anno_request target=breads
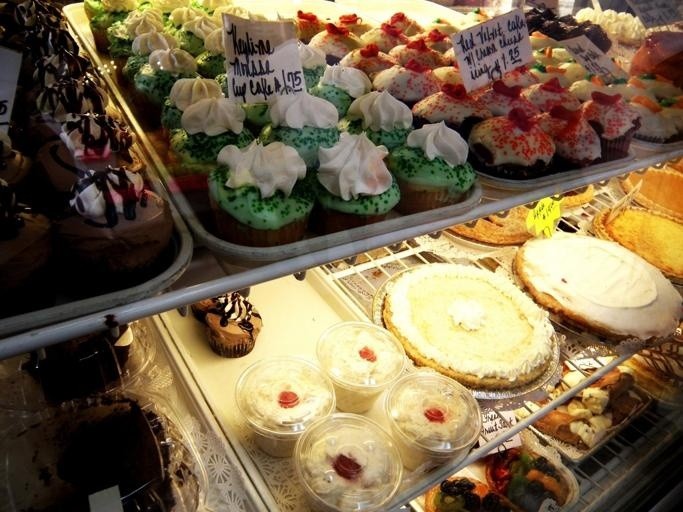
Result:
[524,369,652,462]
[596,205,683,284]
[558,185,595,208]
[619,340,683,402]
[444,202,539,247]
[619,168,683,219]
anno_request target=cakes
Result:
[316,321,410,391]
[414,66,642,179]
[432,10,491,35]
[20,324,142,400]
[22,396,184,511]
[284,10,427,97]
[190,289,263,359]
[528,29,554,52]
[629,31,682,77]
[514,231,683,341]
[530,46,599,105]
[576,6,648,46]
[296,412,403,512]
[383,264,552,388]
[237,359,334,439]
[524,4,612,52]
[388,372,481,459]
[600,69,683,145]
[426,28,470,68]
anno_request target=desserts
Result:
[84,0,476,247]
[1,1,170,280]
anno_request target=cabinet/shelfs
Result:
[0,145,682,512]
